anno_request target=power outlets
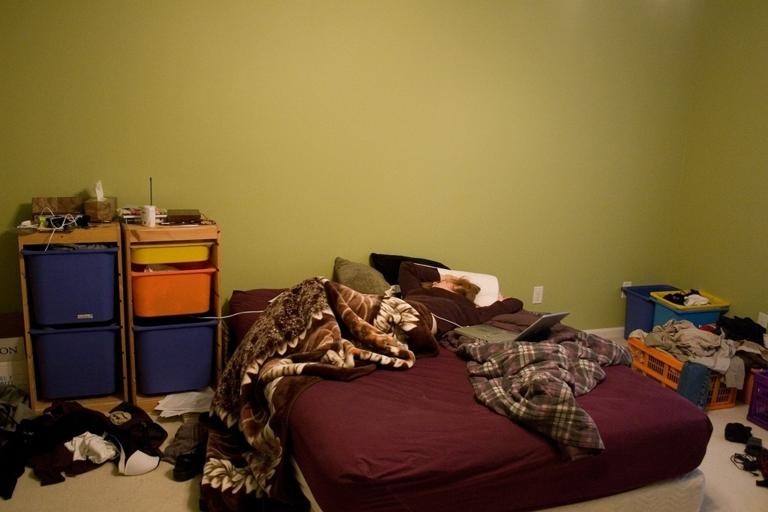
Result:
[532,286,545,304]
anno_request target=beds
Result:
[228,289,713,512]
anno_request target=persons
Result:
[395,262,524,357]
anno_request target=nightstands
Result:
[16,216,131,408]
[123,215,223,408]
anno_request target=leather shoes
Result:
[173,449,206,481]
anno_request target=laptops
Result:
[453,311,571,345]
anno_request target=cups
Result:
[141,205,156,227]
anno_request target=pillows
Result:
[334,256,391,295]
[417,262,500,308]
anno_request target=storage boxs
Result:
[22,246,118,326]
[131,241,214,264]
[746,371,768,433]
[29,325,120,402]
[622,284,680,340]
[648,288,730,332]
[741,367,765,405]
[133,315,218,395]
[626,333,739,412]
[131,263,216,317]
